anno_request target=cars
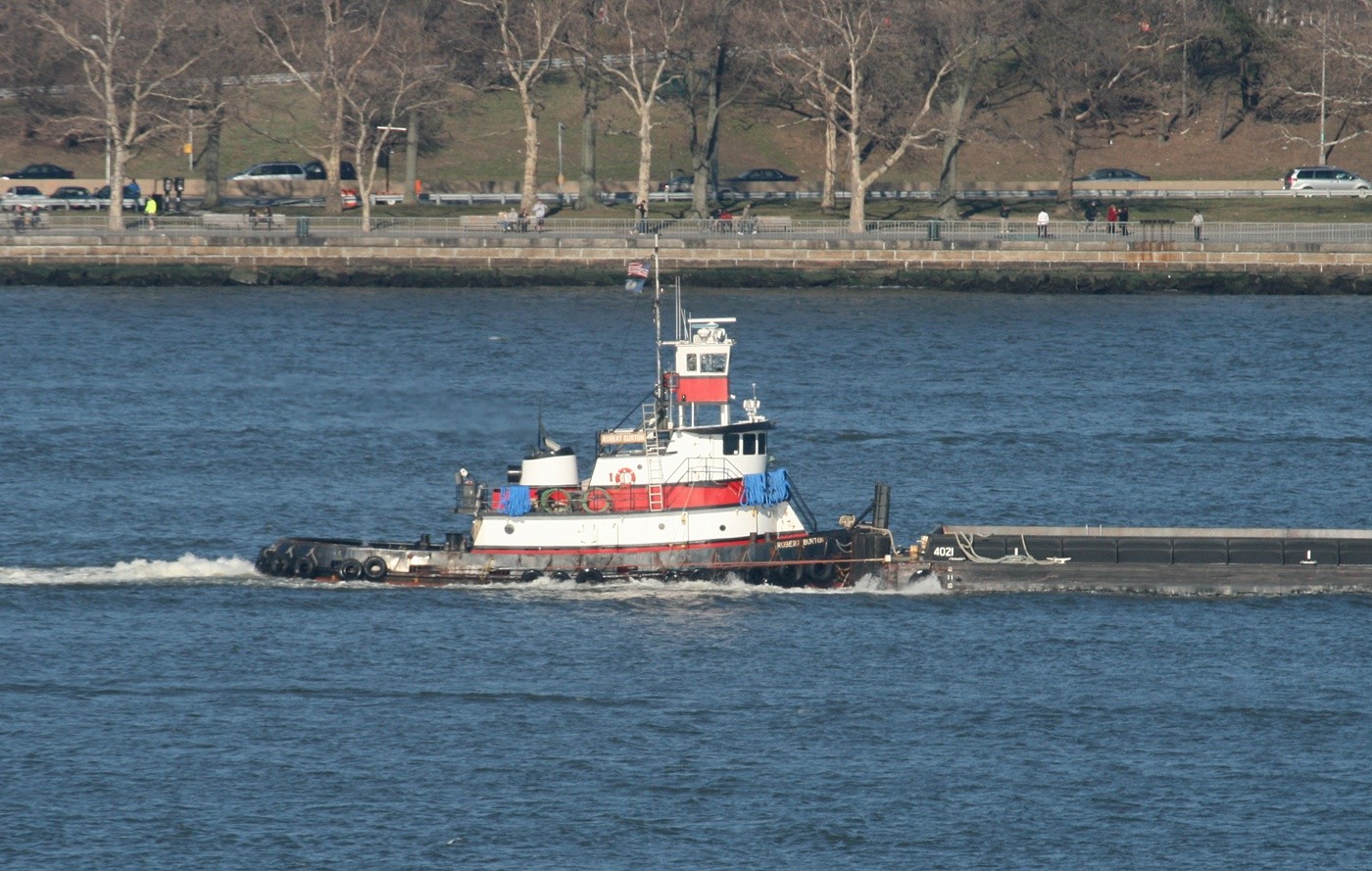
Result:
[0,162,75,180]
[659,174,739,203]
[95,184,144,211]
[302,159,356,180]
[719,167,801,197]
[229,161,308,181]
[2,185,43,196]
[49,186,93,211]
[1072,167,1151,182]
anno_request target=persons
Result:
[709,203,758,235]
[498,200,548,233]
[1000,203,1012,234]
[629,200,647,236]
[143,195,157,231]
[1106,201,1121,235]
[128,177,141,210]
[1084,200,1101,230]
[1191,210,1204,242]
[1036,207,1050,239]
[1114,199,1129,236]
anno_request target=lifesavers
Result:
[575,568,604,584]
[807,558,834,582]
[744,568,768,585]
[717,568,741,584]
[254,557,269,574]
[662,571,678,583]
[778,565,800,585]
[520,570,543,583]
[615,467,636,486]
[271,553,291,577]
[364,556,386,582]
[339,558,362,582]
[549,571,569,581]
[294,557,316,579]
[908,569,932,587]
[688,569,712,583]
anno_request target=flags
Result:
[625,260,653,294]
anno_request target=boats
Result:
[249,228,903,595]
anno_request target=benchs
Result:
[723,216,791,232]
[203,213,286,231]
[460,215,538,233]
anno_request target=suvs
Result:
[1282,165,1372,191]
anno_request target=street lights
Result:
[557,120,567,207]
[89,33,125,186]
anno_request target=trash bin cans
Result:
[928,223,940,240]
[297,216,309,236]
